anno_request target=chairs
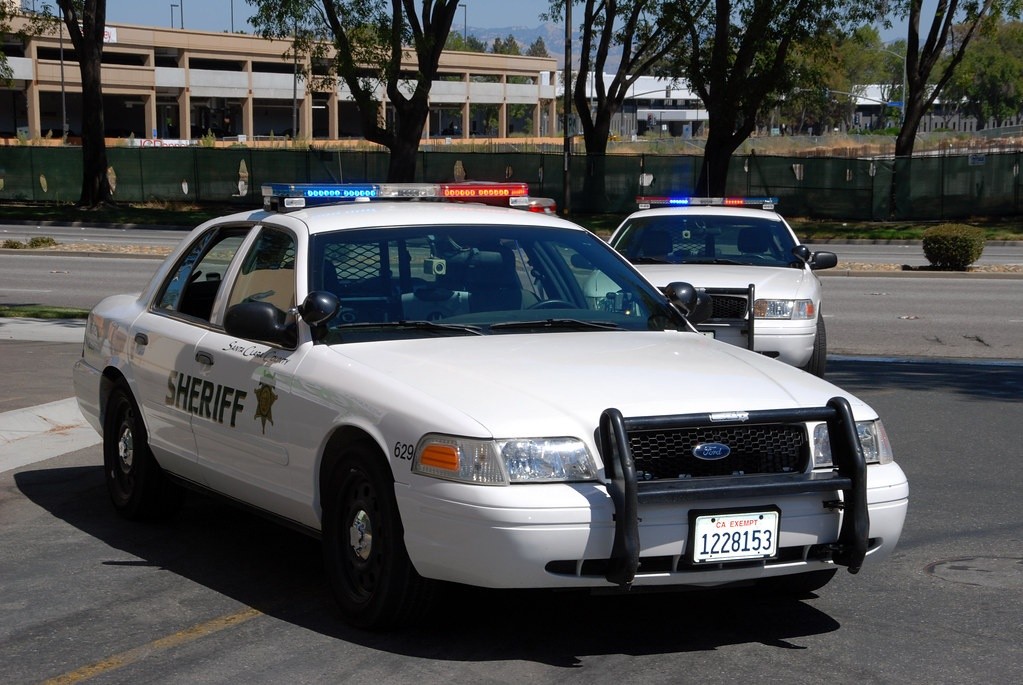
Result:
[283,258,351,329]
[737,227,776,260]
[465,245,541,312]
[632,228,674,260]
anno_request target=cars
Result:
[568,195,839,380]
[73,180,910,635]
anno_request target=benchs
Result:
[179,268,429,324]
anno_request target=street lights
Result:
[170,4,180,29]
[864,48,906,128]
[458,3,468,44]
[659,111,667,138]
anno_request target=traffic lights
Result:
[648,113,653,125]
[652,114,657,126]
[823,87,829,98]
